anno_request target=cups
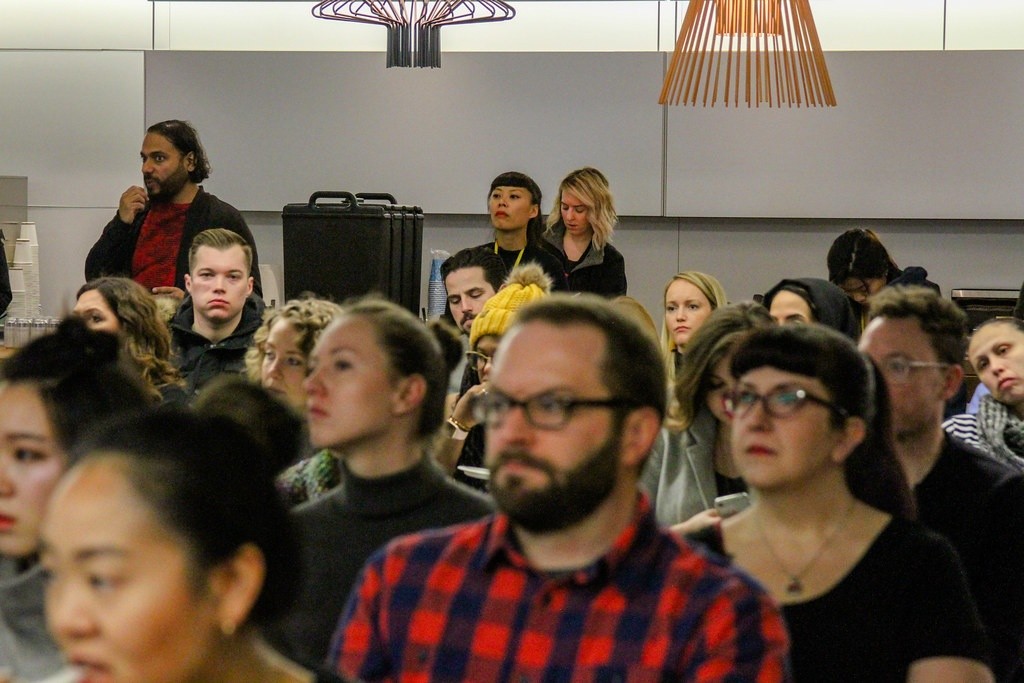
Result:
[13,239,33,317]
[7,268,26,318]
[428,259,447,317]
[20,222,39,319]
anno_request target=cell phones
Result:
[715,493,750,519]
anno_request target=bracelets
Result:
[450,416,472,432]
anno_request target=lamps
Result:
[659,0,839,107]
[311,0,515,70]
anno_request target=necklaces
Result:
[749,507,852,597]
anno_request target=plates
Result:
[457,464,491,480]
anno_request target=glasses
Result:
[471,389,647,430]
[875,356,956,385]
[465,348,496,371]
[722,386,850,419]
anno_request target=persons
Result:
[659,270,726,378]
[284,299,495,661]
[439,245,507,394]
[324,290,790,683]
[434,262,553,480]
[941,316,1024,473]
[73,276,187,415]
[43,373,348,683]
[245,298,344,411]
[0,315,149,683]
[479,172,569,294]
[637,300,776,529]
[84,120,263,303]
[686,322,996,683]
[759,277,856,339]
[827,228,942,334]
[164,228,264,398]
[542,167,628,301]
[857,285,1024,648]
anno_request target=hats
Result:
[470,262,553,351]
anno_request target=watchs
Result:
[444,420,469,441]
